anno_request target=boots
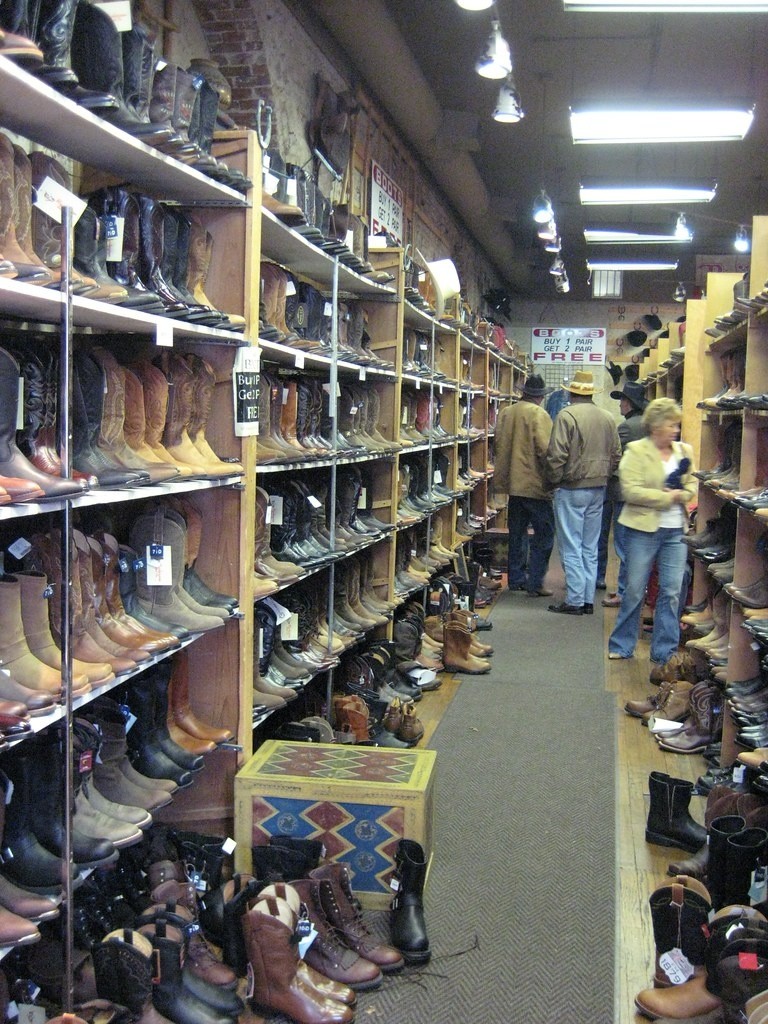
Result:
[0,0,768,1024]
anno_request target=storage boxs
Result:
[483,526,536,572]
[232,741,440,909]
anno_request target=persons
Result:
[601,381,651,608]
[542,371,622,615]
[488,371,554,597]
[609,395,697,669]
[558,479,612,591]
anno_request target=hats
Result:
[560,370,604,395]
[415,245,462,321]
[514,374,556,397]
[610,381,652,411]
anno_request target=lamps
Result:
[672,281,688,303]
[554,269,573,293]
[564,92,758,147]
[733,223,750,254]
[491,75,526,124]
[549,253,567,277]
[583,253,682,270]
[542,233,562,253]
[532,187,556,224]
[472,20,516,81]
[562,0,768,13]
[579,219,694,246]
[580,182,720,208]
[673,211,692,240]
[536,216,558,239]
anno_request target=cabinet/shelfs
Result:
[0,53,768,1024]
